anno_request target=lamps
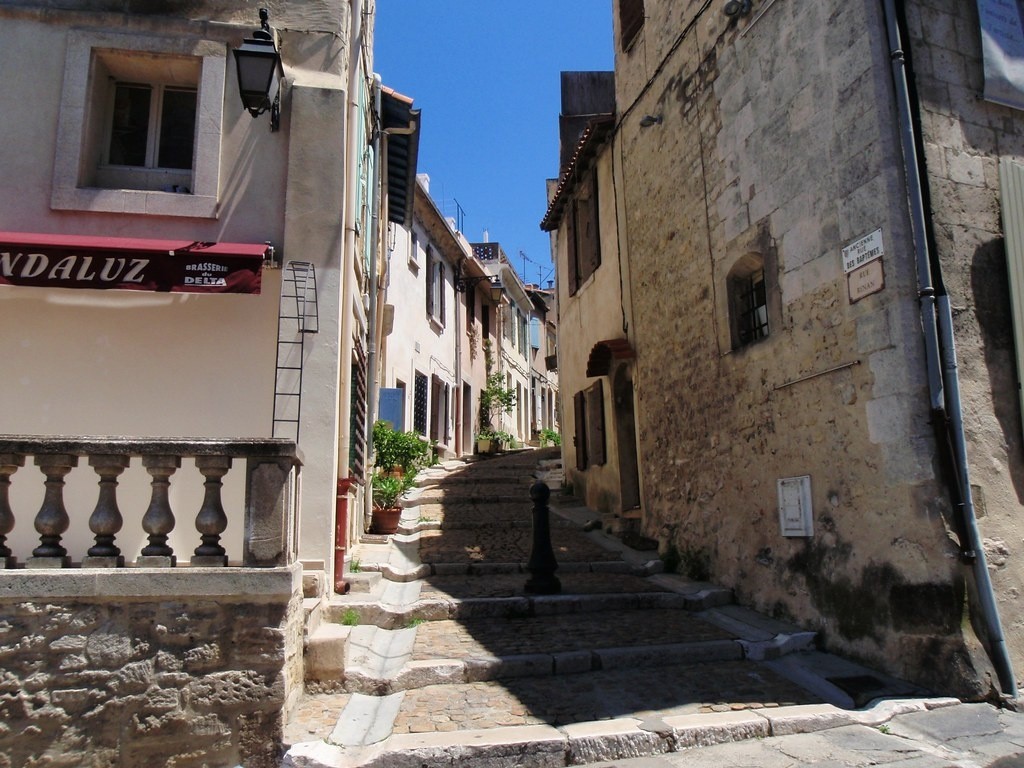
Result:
[456,273,508,308]
[639,113,663,128]
[231,7,286,133]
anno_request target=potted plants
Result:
[528,429,562,448]
[369,421,443,535]
[475,368,520,454]
